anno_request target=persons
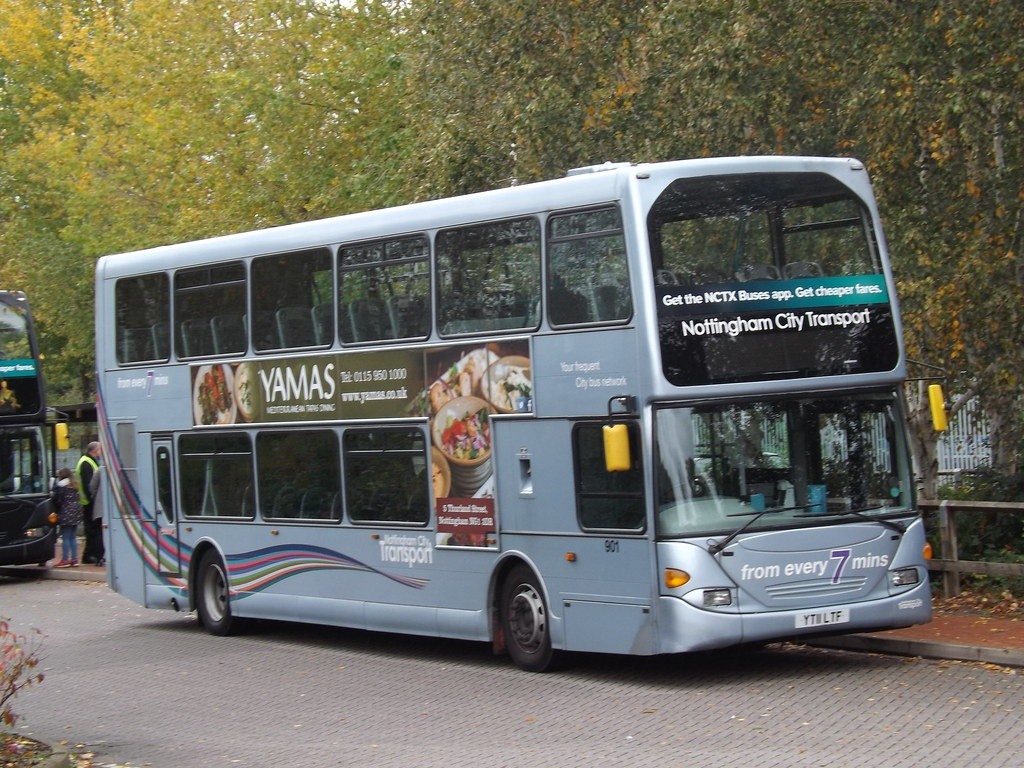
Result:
[51,467,83,568]
[88,464,106,567]
[74,441,106,564]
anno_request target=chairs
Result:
[242,476,427,518]
[123,261,853,360]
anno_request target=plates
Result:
[431,396,498,468]
[479,355,531,413]
[193,364,237,425]
[431,446,452,515]
[234,362,255,419]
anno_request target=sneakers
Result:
[53,561,71,568]
[82,555,105,563]
[71,559,79,567]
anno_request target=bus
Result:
[0,289,72,573]
[95,155,951,673]
